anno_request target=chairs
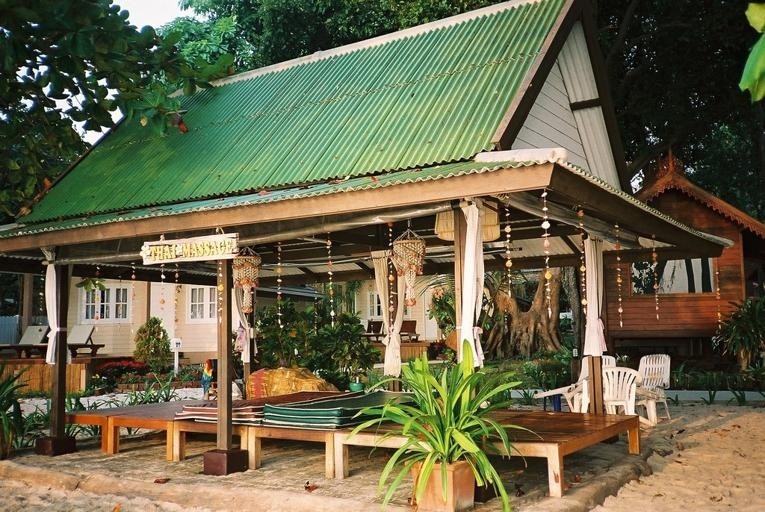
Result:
[562,354,672,440]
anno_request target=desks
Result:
[65,400,204,463]
[173,421,247,463]
[247,426,333,478]
[333,409,641,499]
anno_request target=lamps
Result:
[392,218,426,307]
[232,245,262,314]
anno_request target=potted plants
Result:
[346,339,544,512]
